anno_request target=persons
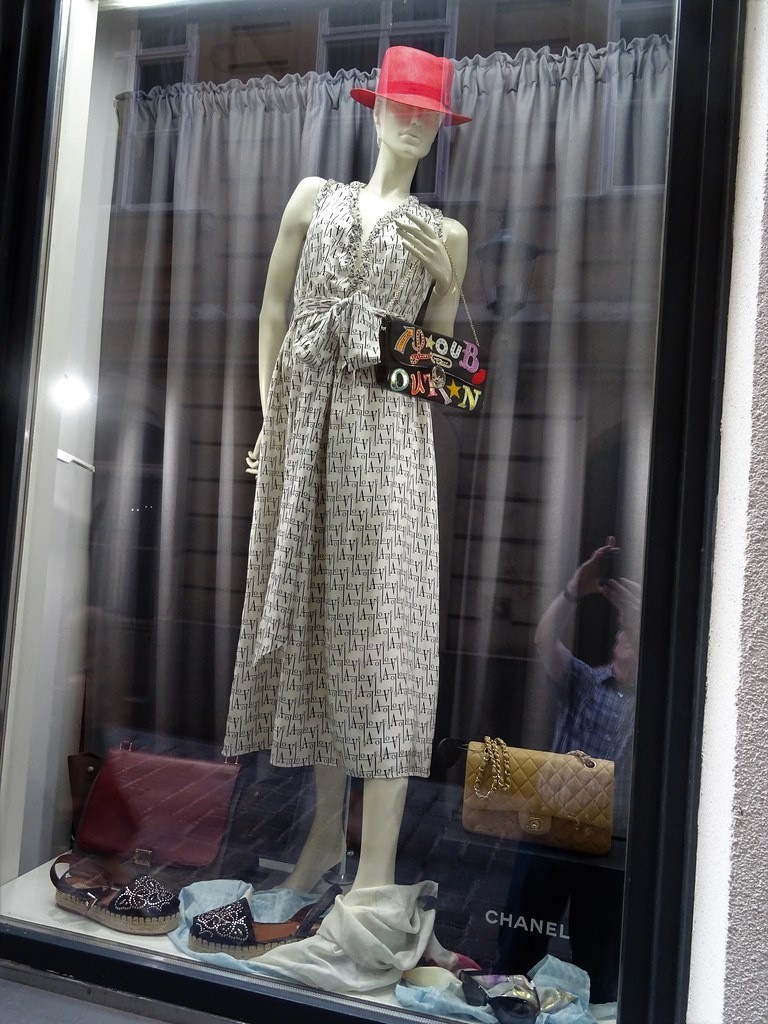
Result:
[220,47,475,898]
[495,537,642,1009]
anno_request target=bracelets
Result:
[562,586,581,603]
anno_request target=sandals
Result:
[187,883,343,962]
[51,852,183,934]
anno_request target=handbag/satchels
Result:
[374,249,490,416]
[461,735,617,855]
[76,741,243,869]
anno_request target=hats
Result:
[349,43,475,126]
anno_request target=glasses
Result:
[460,970,541,1024]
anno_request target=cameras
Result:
[597,550,620,586]
